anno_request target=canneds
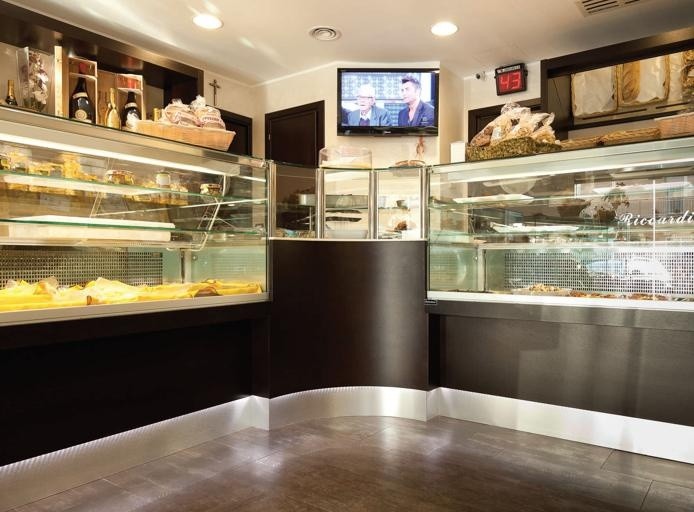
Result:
[200,182,222,206]
[156,172,190,205]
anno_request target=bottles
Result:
[104,87,121,130]
[69,63,95,124]
[199,183,222,204]
[104,169,135,199]
[155,171,171,190]
[153,107,161,122]
[120,77,141,127]
[4,79,19,107]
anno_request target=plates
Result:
[592,182,693,199]
[509,286,573,296]
[452,193,535,209]
[492,224,581,232]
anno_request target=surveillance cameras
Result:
[476,71,486,82]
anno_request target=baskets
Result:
[559,136,601,152]
[137,120,237,151]
[602,127,659,147]
[656,112,694,140]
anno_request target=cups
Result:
[378,195,386,208]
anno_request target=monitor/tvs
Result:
[336,68,439,137]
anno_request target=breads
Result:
[394,159,424,167]
[527,281,694,302]
[1,276,263,313]
[471,124,556,149]
[163,97,226,130]
[0,144,155,204]
[681,49,694,100]
[621,61,641,99]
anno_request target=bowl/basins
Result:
[329,229,369,239]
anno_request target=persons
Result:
[398,75,435,127]
[347,84,392,126]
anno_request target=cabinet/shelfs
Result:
[427,136,694,313]
[268,158,426,241]
[1,102,276,326]
